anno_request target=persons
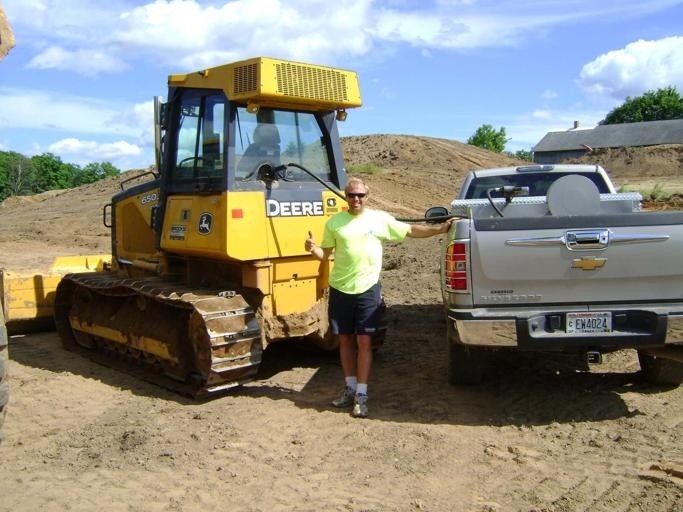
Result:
[304,176,461,418]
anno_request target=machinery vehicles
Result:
[2,56,390,399]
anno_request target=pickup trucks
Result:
[424,162,683,388]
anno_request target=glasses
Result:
[347,193,365,198]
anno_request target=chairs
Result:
[237,124,279,176]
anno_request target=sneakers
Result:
[332,386,369,417]
